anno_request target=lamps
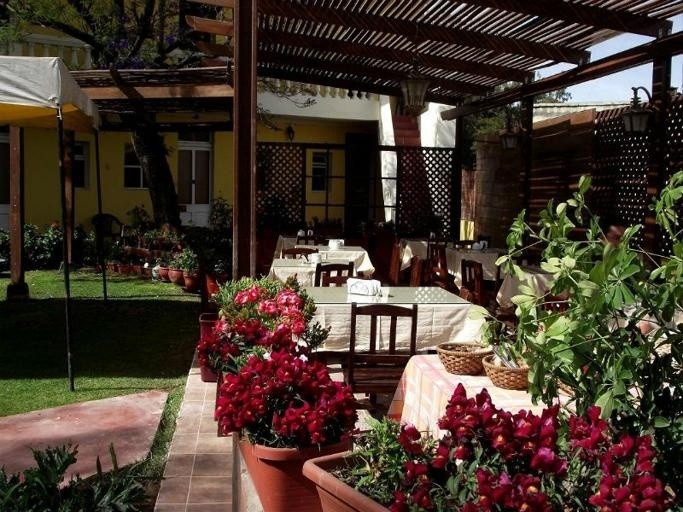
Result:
[397,25,431,117]
[620,85,659,139]
[499,121,521,155]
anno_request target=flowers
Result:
[390,382,669,512]
[189,279,359,443]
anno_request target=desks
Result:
[400,353,579,429]
[264,226,368,288]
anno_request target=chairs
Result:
[91,214,125,244]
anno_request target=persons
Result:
[591,217,630,268]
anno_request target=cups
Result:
[381,288,389,297]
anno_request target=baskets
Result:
[437,342,493,374]
[482,356,531,388]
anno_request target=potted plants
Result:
[107,225,200,290]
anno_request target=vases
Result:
[228,431,388,512]
